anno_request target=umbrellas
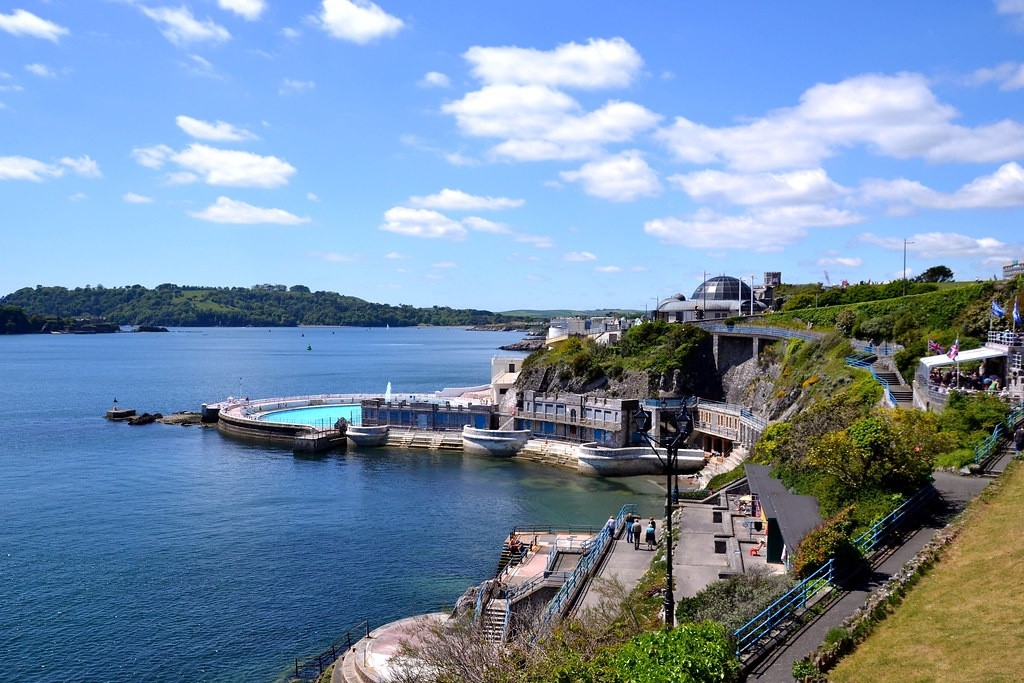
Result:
[740,495,751,501]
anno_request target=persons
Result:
[649,517,656,530]
[740,504,747,513]
[869,339,874,350]
[645,525,655,550]
[996,329,1022,346]
[931,366,1007,396]
[871,276,955,285]
[508,535,519,557]
[631,519,641,550]
[709,490,714,495]
[753,541,765,551]
[625,513,634,543]
[608,516,615,537]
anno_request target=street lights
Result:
[633,397,695,633]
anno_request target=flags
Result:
[929,340,945,354]
[1013,302,1021,324]
[947,342,959,361]
[992,300,1005,319]
